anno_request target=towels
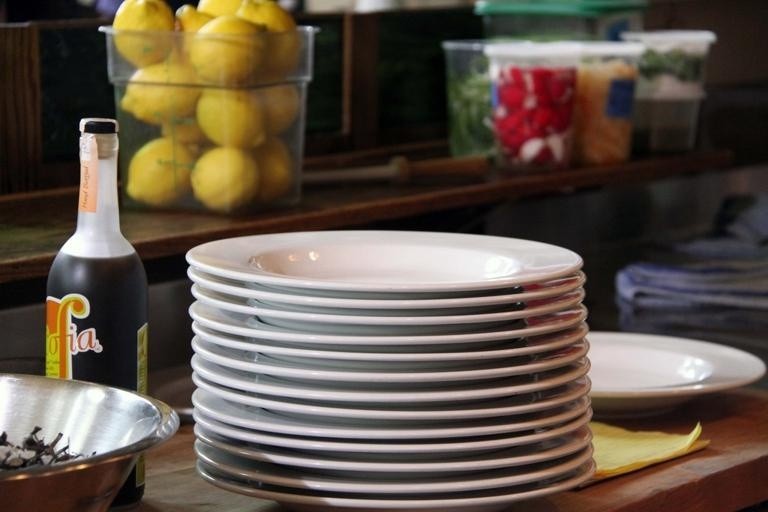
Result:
[614,196,768,315]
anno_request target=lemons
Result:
[111,0,305,215]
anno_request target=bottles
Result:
[43,115,151,510]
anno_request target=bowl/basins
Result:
[1,371,181,512]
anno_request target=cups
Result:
[439,26,717,171]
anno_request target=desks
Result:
[134,386,768,510]
[0,141,768,285]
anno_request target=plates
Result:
[583,328,767,418]
[186,227,598,511]
